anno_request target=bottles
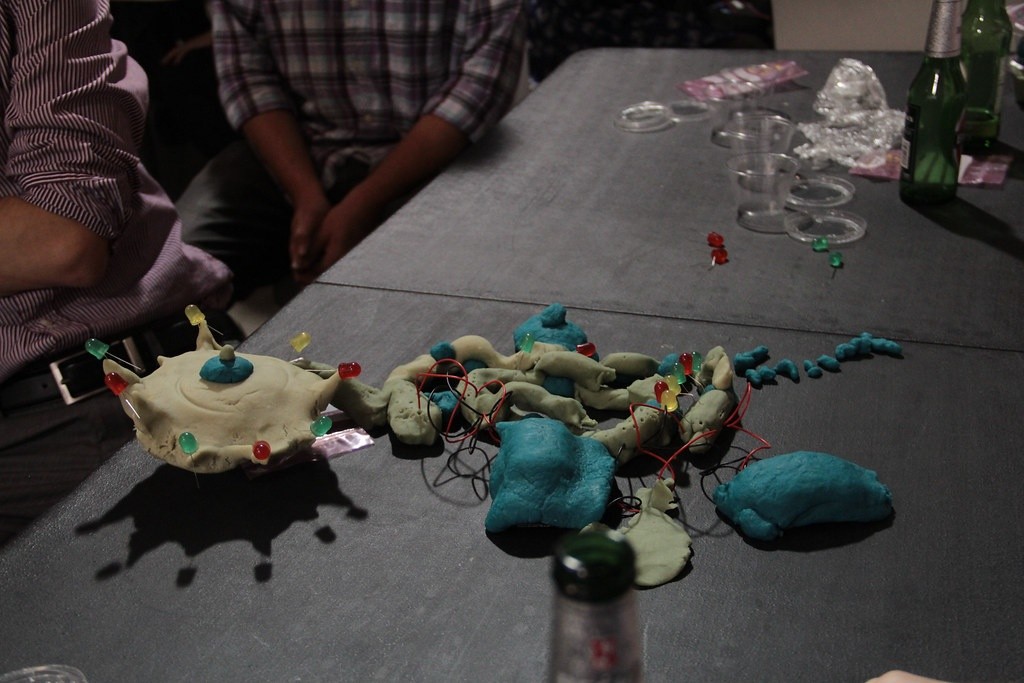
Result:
[899,0,966,209]
[547,534,645,682]
[961,0,1013,157]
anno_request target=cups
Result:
[703,81,757,147]
[725,153,798,216]
[725,116,797,157]
[0,664,88,683]
[731,64,778,119]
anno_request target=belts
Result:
[0,322,183,410]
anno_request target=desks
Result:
[0,47,1024,683]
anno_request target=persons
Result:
[175,0,532,301]
[0,0,247,552]
